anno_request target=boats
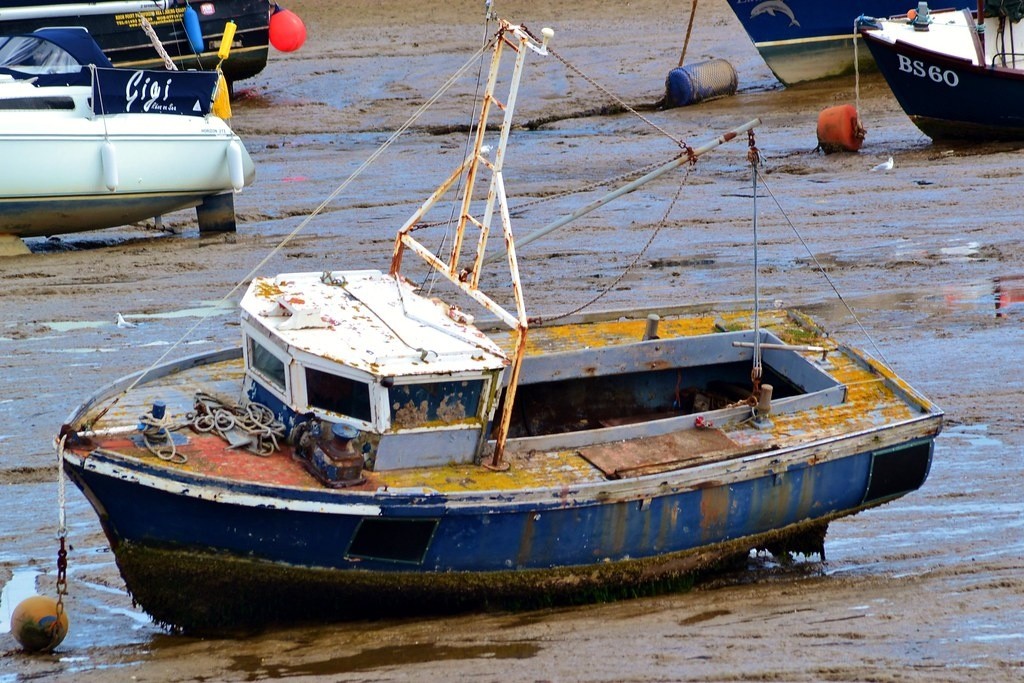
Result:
[0,0,269,237]
[861,3,1020,146]
[728,0,983,88]
[53,1,942,636]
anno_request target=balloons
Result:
[269,4,306,52]
